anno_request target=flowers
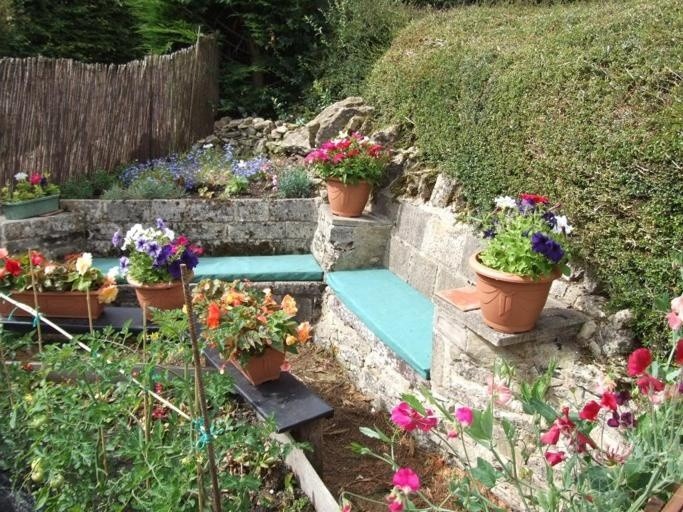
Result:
[0,168,61,202]
[336,288,683,512]
[467,190,575,284]
[306,127,393,189]
[191,276,309,372]
[0,248,118,304]
[111,217,204,287]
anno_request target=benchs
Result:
[90,253,322,325]
[313,267,434,411]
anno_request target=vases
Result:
[468,250,561,335]
[325,177,371,217]
[1,194,60,220]
[229,349,282,386]
[125,269,193,322]
[0,291,105,321]
